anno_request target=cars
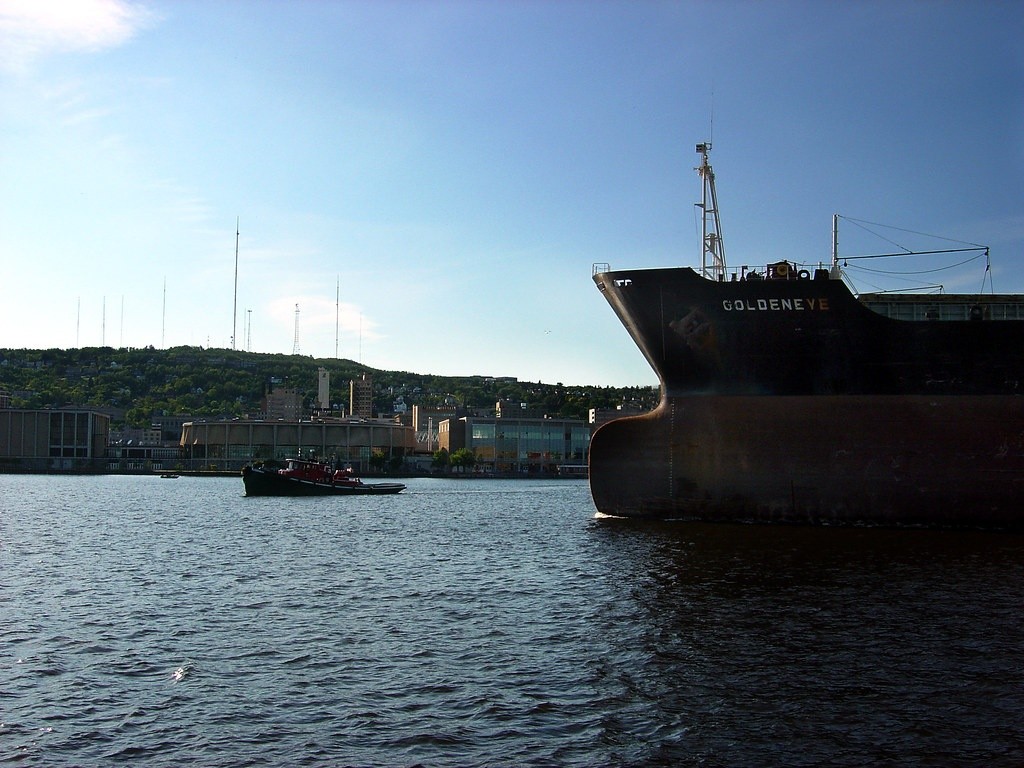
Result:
[416,468,430,473]
[430,468,446,475]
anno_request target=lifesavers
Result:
[969,305,984,320]
[777,264,788,276]
[797,270,811,281]
[549,464,556,471]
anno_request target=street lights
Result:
[547,432,551,461]
[526,432,529,458]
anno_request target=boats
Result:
[242,447,406,497]
[552,464,589,479]
[588,141,1024,521]
[161,473,179,478]
